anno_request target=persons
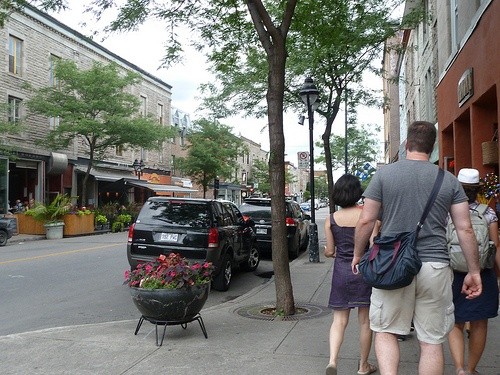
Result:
[351,121,482,375]
[3,199,29,214]
[325,168,500,375]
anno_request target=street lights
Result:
[297,75,324,264]
[132,158,145,206]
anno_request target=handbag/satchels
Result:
[358,231,422,290]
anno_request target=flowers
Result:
[121,253,216,292]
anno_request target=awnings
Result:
[77,166,198,196]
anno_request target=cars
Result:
[299,193,330,211]
[0,215,13,247]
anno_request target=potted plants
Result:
[16,202,47,235]
[95,215,108,226]
[65,208,96,236]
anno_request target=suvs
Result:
[126,196,261,291]
[238,199,314,260]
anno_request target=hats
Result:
[457,168,485,190]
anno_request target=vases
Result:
[129,282,211,325]
[44,219,64,239]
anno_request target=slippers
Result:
[326,365,337,375]
[356,364,377,375]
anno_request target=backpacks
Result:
[447,203,496,272]
